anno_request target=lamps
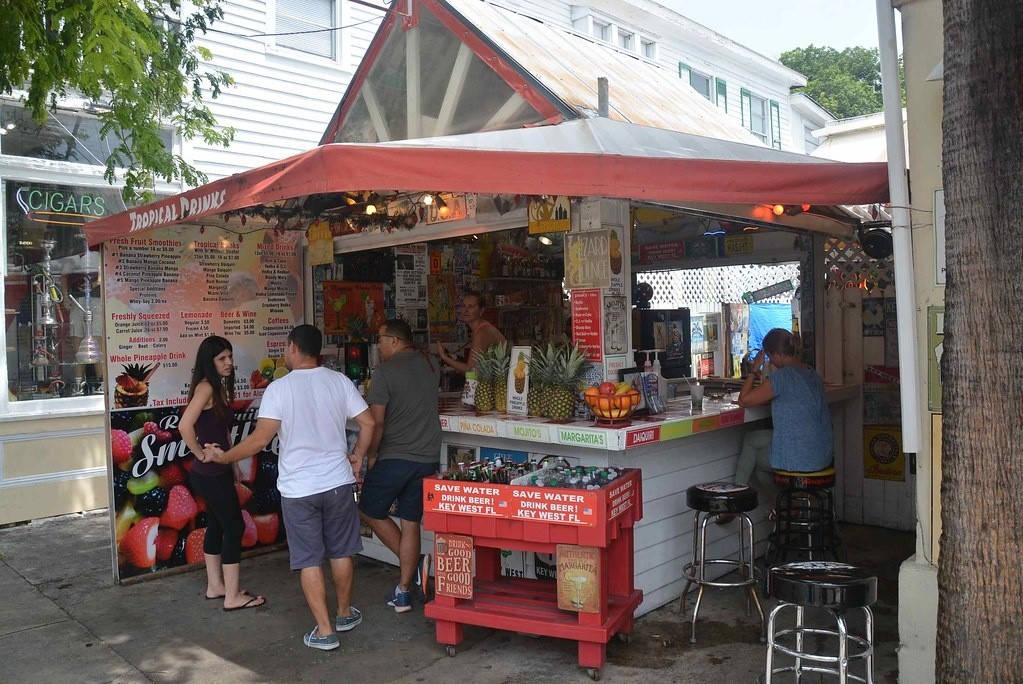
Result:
[347,217,359,231]
[538,233,559,246]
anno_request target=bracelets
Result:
[751,371,757,376]
[198,453,205,462]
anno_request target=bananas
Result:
[614,382,632,394]
[594,407,628,417]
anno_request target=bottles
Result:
[351,366,372,401]
[428,458,624,515]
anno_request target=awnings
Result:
[83,116,912,251]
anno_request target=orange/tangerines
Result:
[585,388,640,409]
[259,358,289,379]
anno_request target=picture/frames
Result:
[619,368,650,416]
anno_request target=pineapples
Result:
[113,363,150,409]
[610,228,622,274]
[472,341,511,413]
[515,351,526,394]
[522,341,593,421]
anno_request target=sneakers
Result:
[413,553,432,604]
[385,583,412,612]
[336,606,362,631]
[304,625,340,650]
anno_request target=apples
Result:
[599,382,616,394]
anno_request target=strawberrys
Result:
[250,370,270,389]
[111,422,278,568]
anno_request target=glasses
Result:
[375,333,405,344]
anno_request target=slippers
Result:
[764,509,799,520]
[223,597,265,611]
[205,591,249,600]
[715,515,735,525]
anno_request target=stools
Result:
[680,467,877,684]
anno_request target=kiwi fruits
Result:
[262,367,274,379]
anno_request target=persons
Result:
[557,320,572,346]
[357,319,442,613]
[208,324,376,649]
[717,329,834,524]
[436,293,507,372]
[178,337,267,612]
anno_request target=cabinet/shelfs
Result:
[480,277,560,344]
[423,466,643,681]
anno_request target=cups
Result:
[690,385,706,411]
